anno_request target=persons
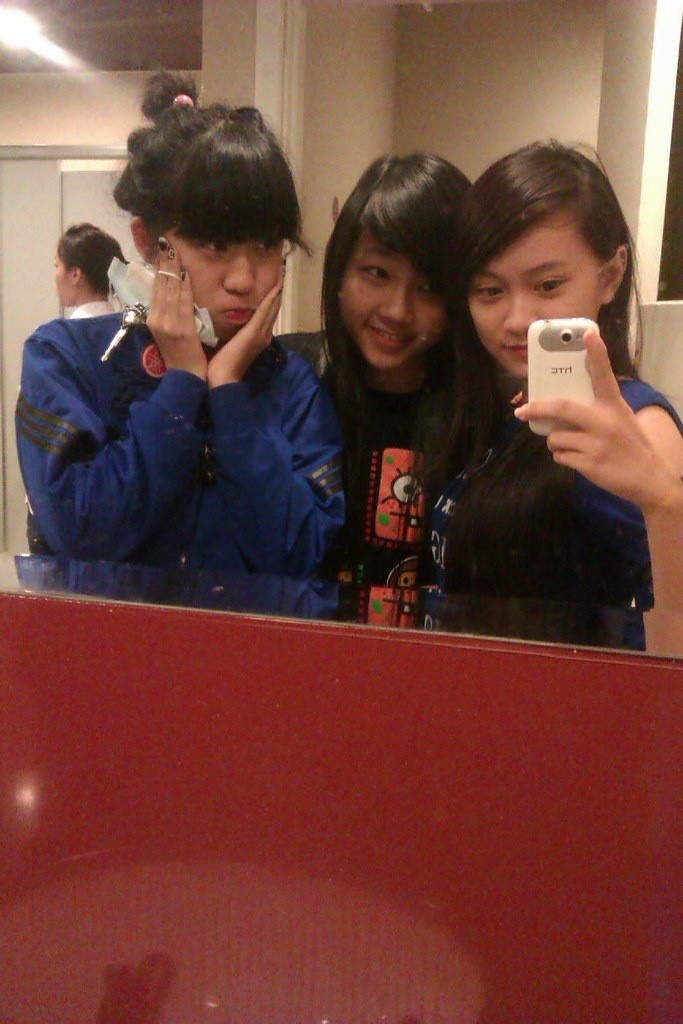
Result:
[14,71,346,580]
[391,142,683,611]
[24,222,129,556]
[274,152,508,591]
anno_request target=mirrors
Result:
[0,0,682,664]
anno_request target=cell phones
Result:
[527,318,601,438]
